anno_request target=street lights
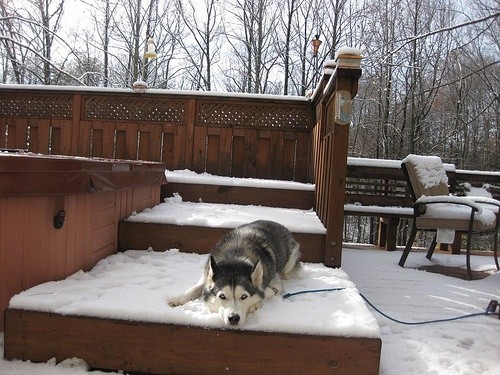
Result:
[311,34,322,82]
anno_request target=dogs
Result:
[168,220,302,326]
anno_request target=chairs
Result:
[398,154,500,279]
